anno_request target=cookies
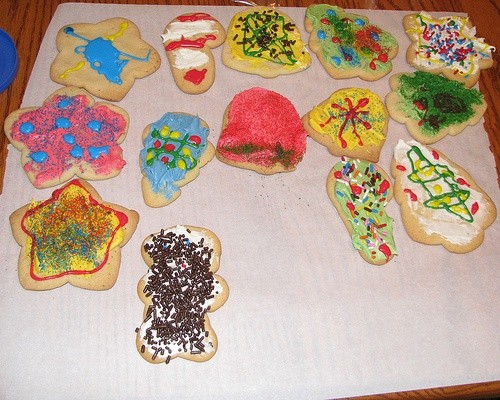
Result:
[49,14,159,103]
[220,7,312,80]
[3,85,131,188]
[216,86,306,175]
[300,88,391,163]
[10,179,141,292]
[161,12,226,95]
[385,70,488,143]
[134,224,230,363]
[326,159,400,266]
[404,11,496,88]
[139,112,216,210]
[391,139,497,254]
[304,3,399,81]
